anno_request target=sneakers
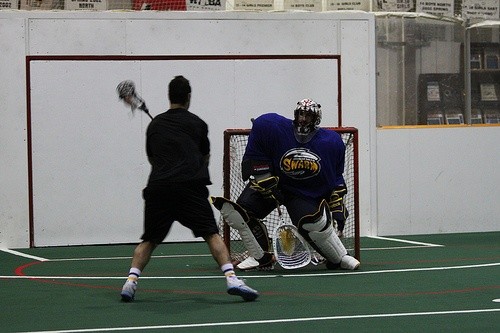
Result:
[226,280,258,300]
[120,280,137,302]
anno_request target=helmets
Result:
[293,98,321,135]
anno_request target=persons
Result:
[210,98,361,269]
[120,74,259,301]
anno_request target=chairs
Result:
[470,54,498,69]
[426,113,499,124]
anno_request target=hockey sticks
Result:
[273,201,311,269]
[115,80,154,120]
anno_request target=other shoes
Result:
[246,261,274,272]
[340,255,359,270]
